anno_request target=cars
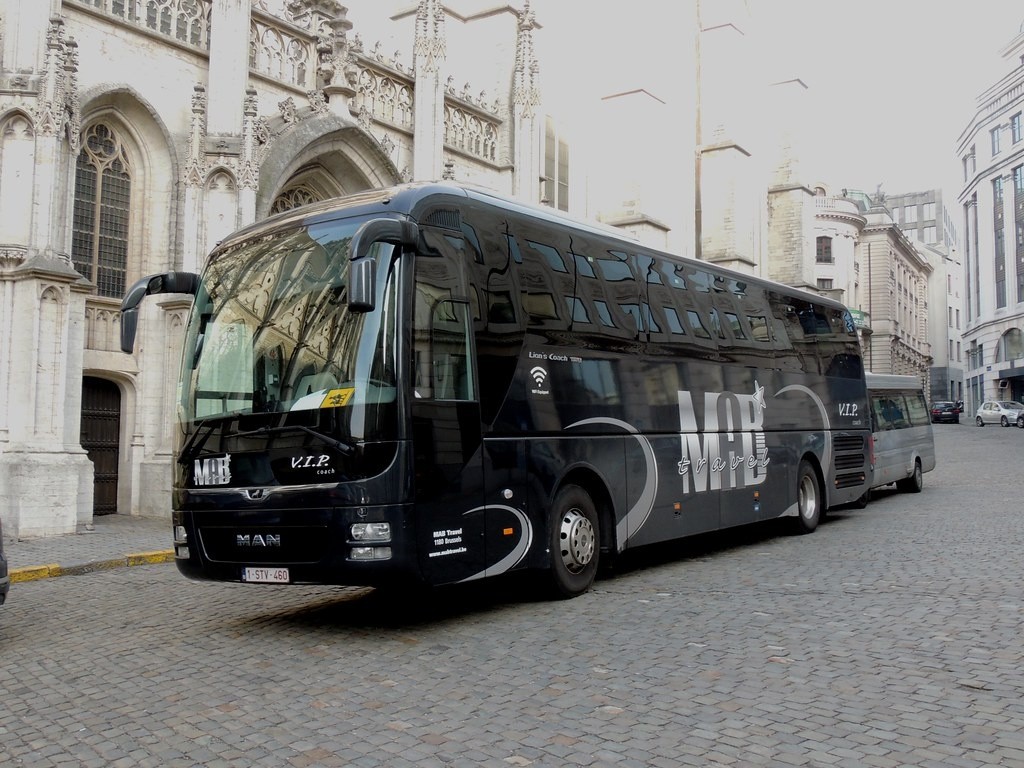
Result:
[974,400,1024,429]
[928,400,961,424]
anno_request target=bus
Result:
[117,180,874,616]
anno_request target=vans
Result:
[861,373,938,495]
